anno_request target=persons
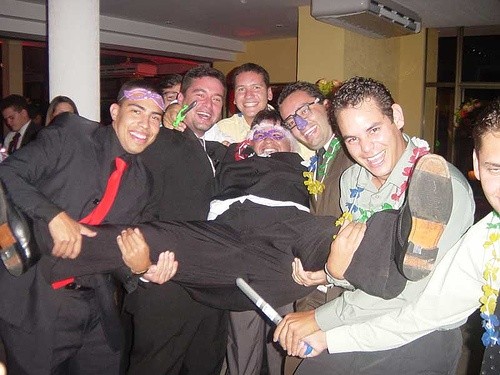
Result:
[26,100,47,126]
[0,94,41,164]
[0,108,455,302]
[160,63,316,375]
[0,75,166,375]
[124,67,227,375]
[44,95,80,126]
[158,71,182,107]
[275,80,357,375]
[270,76,476,375]
[287,106,500,375]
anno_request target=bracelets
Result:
[131,260,152,275]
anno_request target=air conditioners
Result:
[100,62,158,77]
[310,0,422,41]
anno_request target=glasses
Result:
[281,99,323,130]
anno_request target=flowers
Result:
[456,99,486,129]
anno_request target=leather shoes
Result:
[394,154,454,281]
[0,179,43,277]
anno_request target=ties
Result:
[7,132,21,155]
[51,157,127,289]
[315,147,326,181]
